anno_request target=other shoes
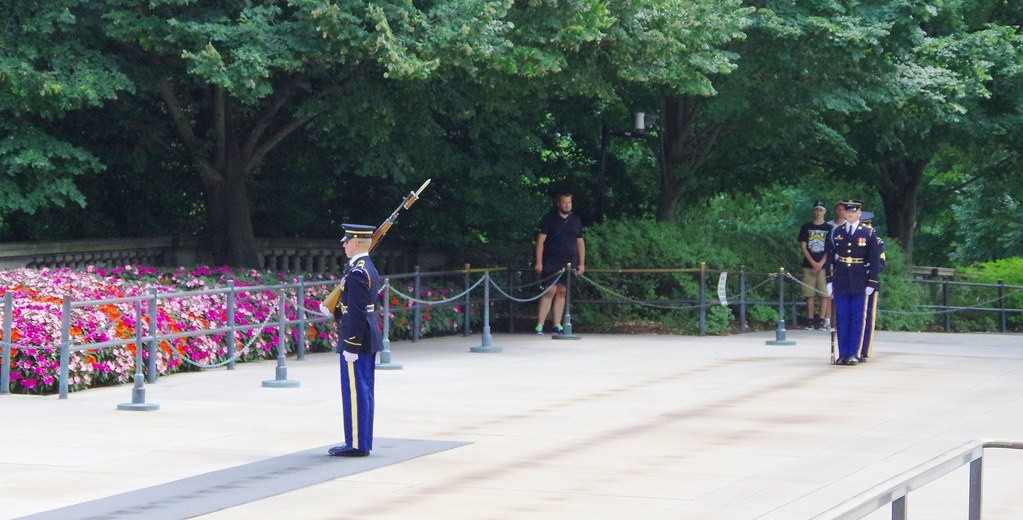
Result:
[804,318,815,330]
[328,444,370,456]
[836,355,859,365]
[819,318,827,331]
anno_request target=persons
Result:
[319,223,383,457]
[798,199,886,365]
[535,190,585,335]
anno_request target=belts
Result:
[838,256,864,263]
[340,303,375,314]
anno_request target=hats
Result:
[813,202,827,211]
[842,198,864,211]
[835,200,847,210]
[861,211,874,225]
[340,223,376,242]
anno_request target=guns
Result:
[829,263,836,365]
[319,178,432,318]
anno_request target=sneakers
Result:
[552,324,564,335]
[535,323,544,334]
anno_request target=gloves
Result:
[320,305,334,320]
[342,350,358,362]
[865,286,875,295]
[826,283,833,297]
